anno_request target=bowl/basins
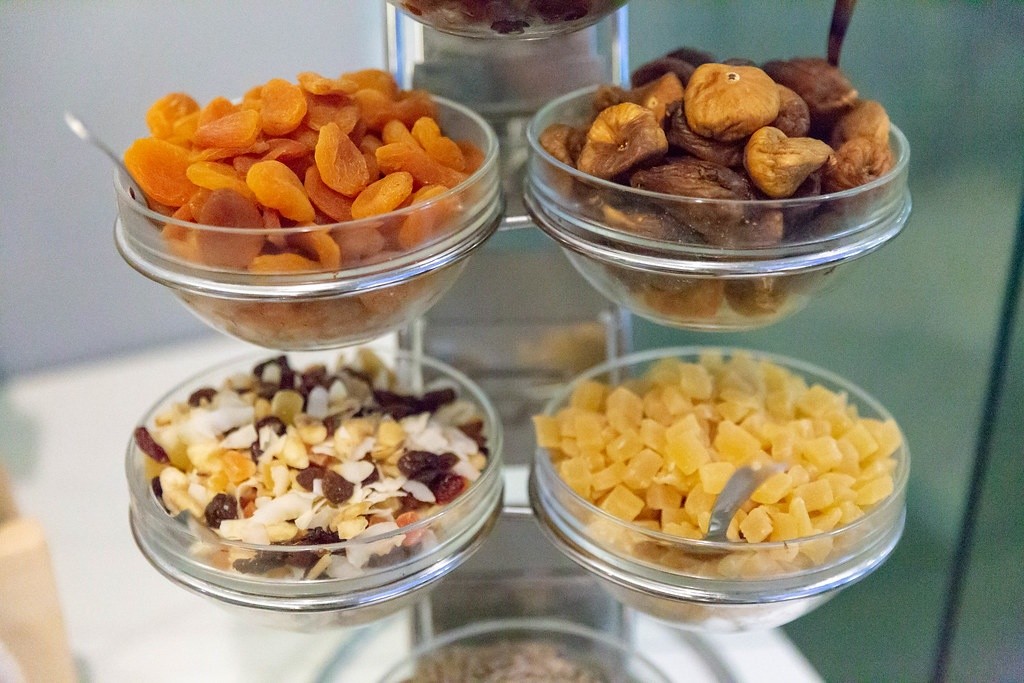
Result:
[527,343,912,632]
[383,0,630,43]
[370,618,675,683]
[112,85,505,352]
[127,349,504,634]
[519,86,916,335]
